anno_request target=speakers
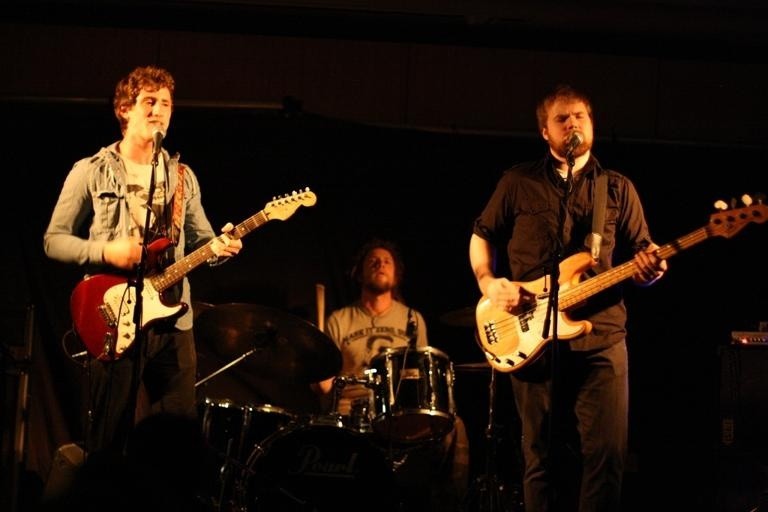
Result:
[715,349,768,458]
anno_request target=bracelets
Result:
[476,266,492,280]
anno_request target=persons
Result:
[468,86,669,512]
[310,244,470,478]
[43,66,244,435]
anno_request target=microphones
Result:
[149,125,168,165]
[562,130,584,158]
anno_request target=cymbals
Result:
[438,305,478,328]
[192,304,342,386]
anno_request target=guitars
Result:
[70,187,319,362]
[476,193,765,373]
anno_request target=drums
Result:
[234,404,296,462]
[200,398,244,461]
[370,344,455,447]
[230,411,405,512]
